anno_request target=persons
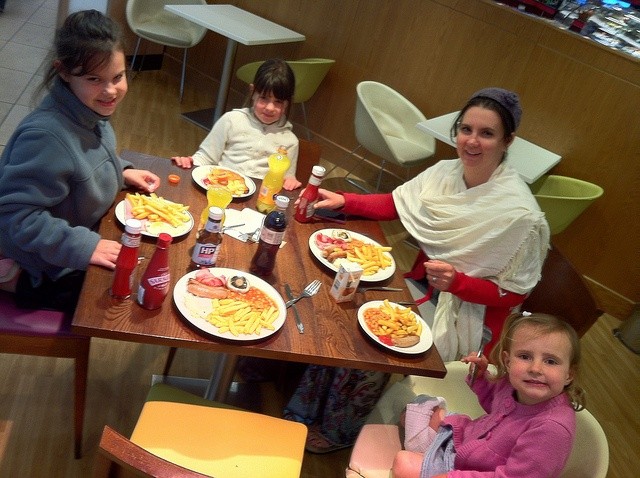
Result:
[389,307,587,477]
[280,87,545,450]
[170,57,302,191]
[1,7,161,316]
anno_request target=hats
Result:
[471,88,523,132]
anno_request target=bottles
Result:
[569,11,589,34]
[137,232,173,311]
[111,219,141,299]
[256,145,291,213]
[294,166,326,223]
[189,206,224,270]
[196,177,233,242]
[249,195,289,277]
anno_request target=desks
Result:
[417,109,562,185]
[163,1,306,133]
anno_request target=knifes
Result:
[284,284,304,334]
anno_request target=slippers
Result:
[305,425,355,454]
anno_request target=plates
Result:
[115,197,195,237]
[173,267,288,343]
[357,300,434,355]
[308,227,397,283]
[192,165,257,199]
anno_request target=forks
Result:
[356,286,402,293]
[469,325,493,386]
[285,279,322,309]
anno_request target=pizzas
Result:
[216,168,243,182]
[363,307,387,335]
[345,239,367,249]
[228,285,280,311]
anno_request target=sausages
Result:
[188,284,228,299]
[328,251,348,263]
[322,244,335,258]
[189,277,227,289]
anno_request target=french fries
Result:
[348,245,394,279]
[125,192,193,228]
[227,178,248,197]
[205,297,280,337]
[379,298,422,340]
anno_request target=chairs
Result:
[532,174,606,237]
[519,243,606,340]
[321,80,436,195]
[234,57,335,141]
[97,380,309,478]
[299,141,320,185]
[343,358,611,478]
[126,1,207,98]
[0,258,92,461]
